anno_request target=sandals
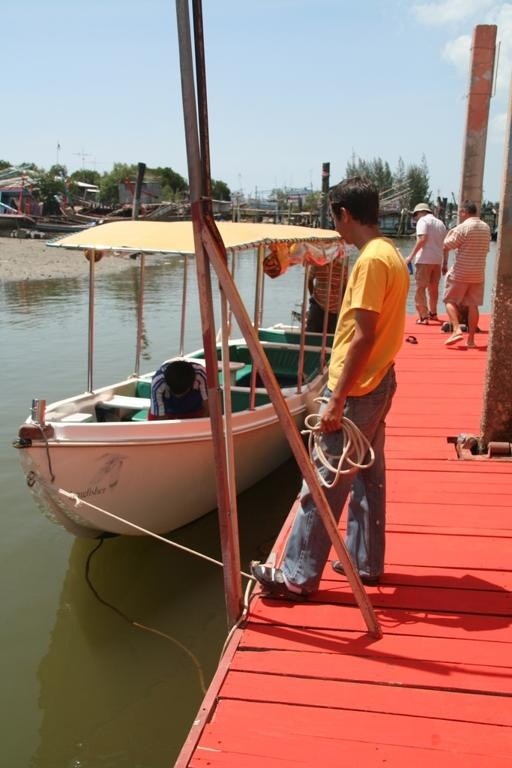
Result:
[251,560,311,605]
[331,557,382,586]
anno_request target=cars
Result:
[0,199,37,234]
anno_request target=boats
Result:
[10,219,345,539]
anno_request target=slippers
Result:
[405,335,419,343]
[444,334,464,346]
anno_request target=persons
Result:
[440,197,493,348]
[302,255,348,335]
[244,173,413,603]
[404,201,449,325]
[145,356,226,421]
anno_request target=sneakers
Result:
[415,316,430,325]
[428,312,439,321]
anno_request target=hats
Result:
[410,201,434,214]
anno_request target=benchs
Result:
[123,330,334,421]
